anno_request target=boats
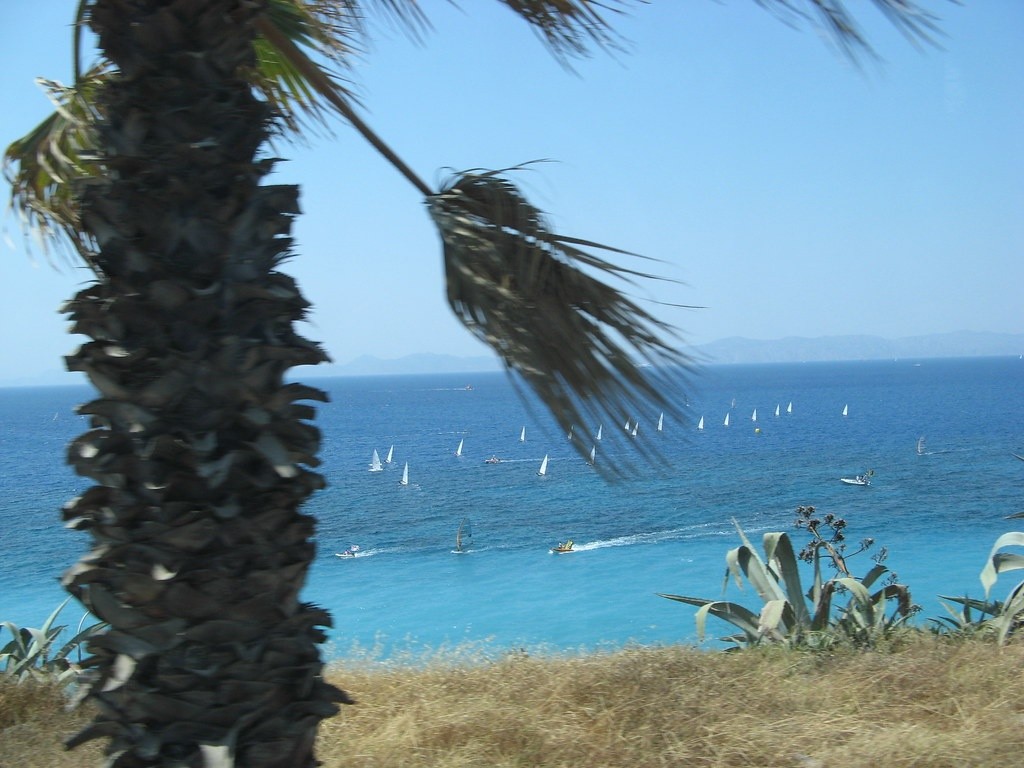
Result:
[552,540,574,553]
[335,552,355,557]
[839,472,870,485]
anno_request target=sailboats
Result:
[367,395,850,487]
[453,519,474,555]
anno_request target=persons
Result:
[345,549,350,555]
[858,474,868,483]
[558,542,562,548]
